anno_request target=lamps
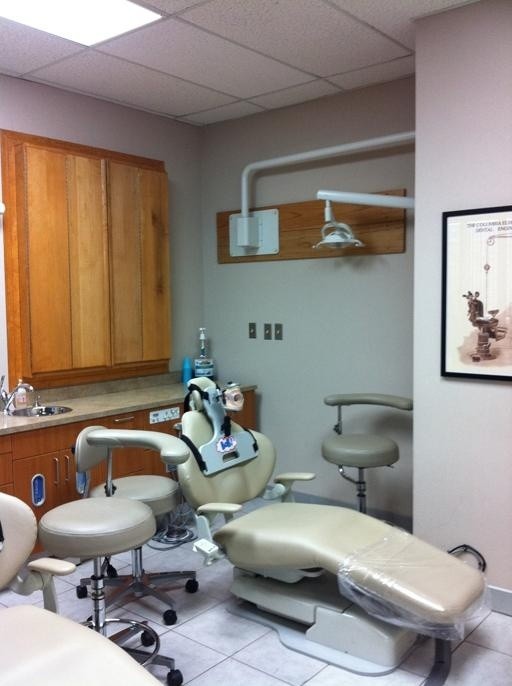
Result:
[311,189,414,248]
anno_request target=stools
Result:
[38,496,184,685]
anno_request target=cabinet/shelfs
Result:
[10,420,102,553]
[110,410,151,478]
[20,140,172,373]
[0,435,15,496]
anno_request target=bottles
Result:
[199,326,209,358]
[182,356,193,385]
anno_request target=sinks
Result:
[11,403,72,418]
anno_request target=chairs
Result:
[322,393,413,513]
[179,376,483,675]
[75,424,197,623]
[0,491,164,686]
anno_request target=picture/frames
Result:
[440,205,512,381]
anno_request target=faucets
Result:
[0,375,34,415]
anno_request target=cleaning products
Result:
[195,326,215,384]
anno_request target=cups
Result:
[195,359,214,378]
[14,392,30,408]
